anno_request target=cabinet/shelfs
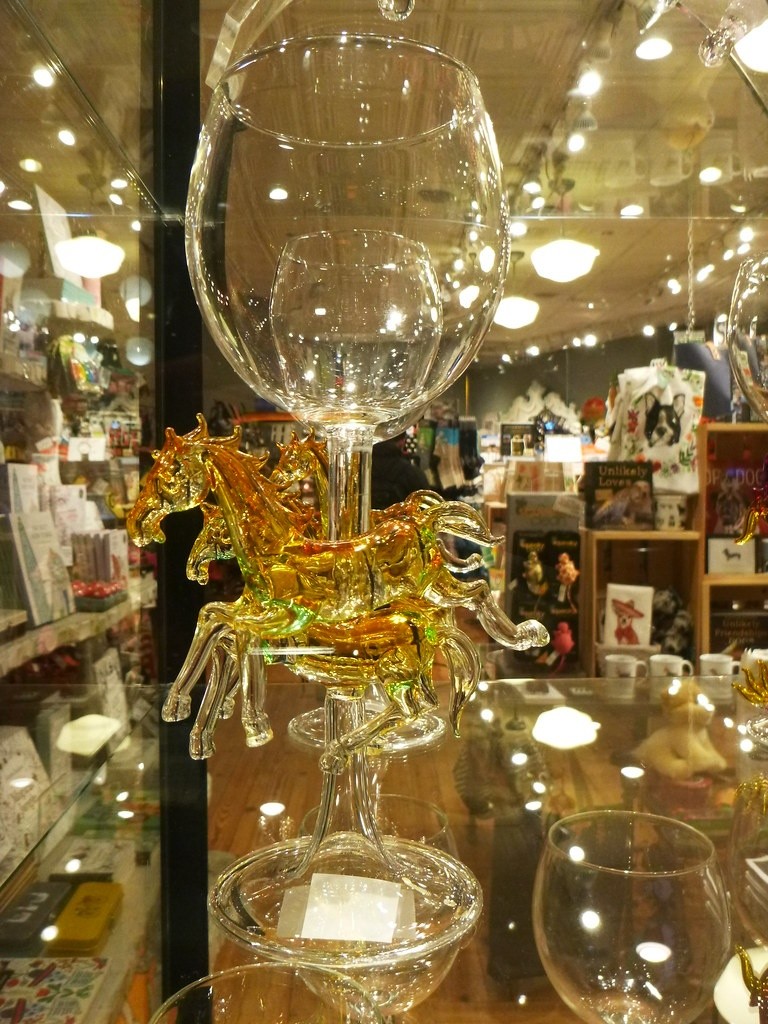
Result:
[585,528,702,677]
[694,421,768,675]
[0,571,163,1023]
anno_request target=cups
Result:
[294,794,461,1024]
[701,653,741,677]
[606,654,648,678]
[150,963,382,1024]
[732,781,768,947]
[726,255,768,426]
[647,654,694,677]
[531,814,728,1024]
[653,495,687,530]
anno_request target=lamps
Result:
[521,0,666,209]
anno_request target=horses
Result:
[124,413,551,777]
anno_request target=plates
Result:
[713,946,768,1024]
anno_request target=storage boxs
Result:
[44,880,123,957]
[506,490,586,670]
[0,881,75,958]
[37,702,71,781]
[595,641,661,677]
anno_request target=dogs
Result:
[611,680,727,778]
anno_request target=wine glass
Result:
[185,36,509,962]
